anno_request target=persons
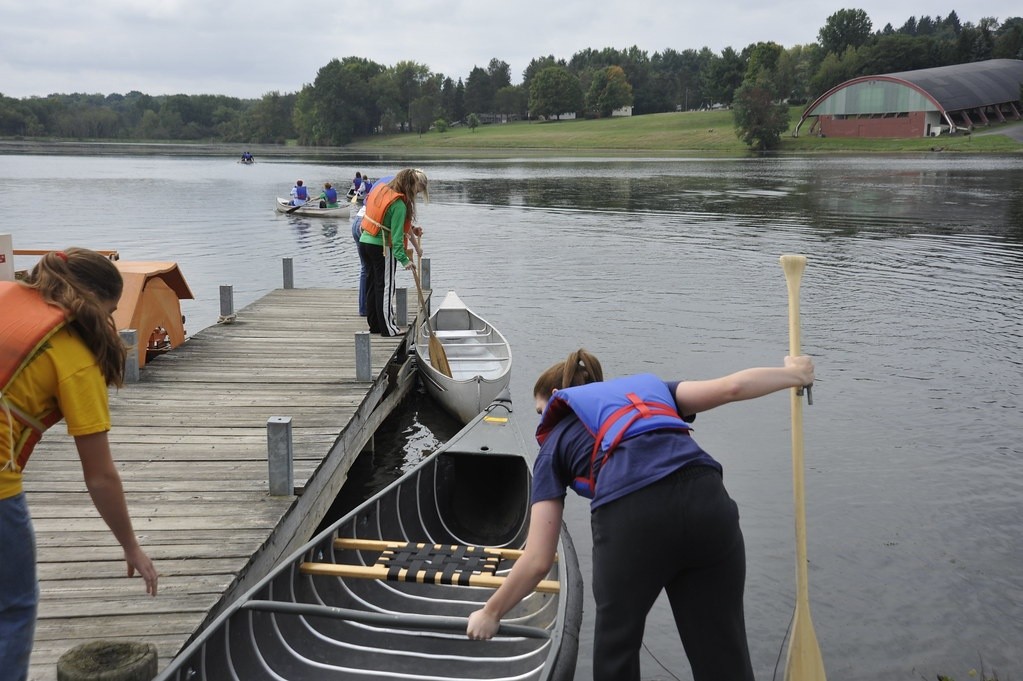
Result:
[352,168,429,317]
[0,245,159,681]
[466,348,816,681]
[359,168,419,337]
[241,151,254,161]
[310,183,339,209]
[352,172,362,195]
[358,175,372,196]
[288,180,310,206]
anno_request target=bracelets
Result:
[412,226,414,229]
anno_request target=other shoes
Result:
[396,329,406,335]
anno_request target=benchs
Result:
[299,537,561,595]
[419,343,505,346]
[422,324,493,338]
[425,357,509,361]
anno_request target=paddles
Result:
[286,197,318,214]
[407,246,454,378]
[414,228,421,278]
[351,192,358,203]
[781,251,829,681]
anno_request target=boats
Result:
[414,289,514,424]
[276,195,358,218]
[346,183,367,201]
[146,383,583,681]
[241,159,254,165]
